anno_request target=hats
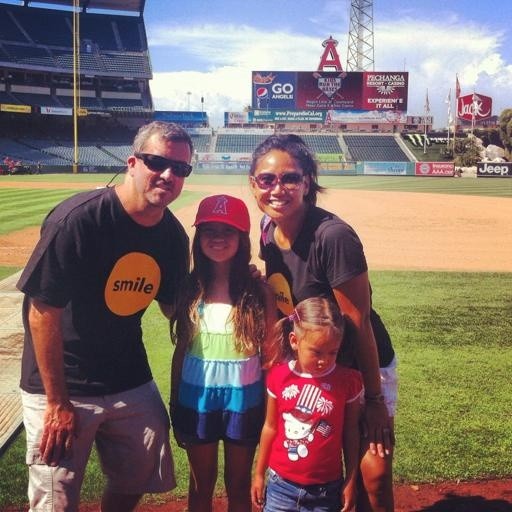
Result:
[192,195,251,233]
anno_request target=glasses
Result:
[254,172,303,188]
[134,152,193,176]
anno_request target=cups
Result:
[253,76,273,109]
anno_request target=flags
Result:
[444,90,450,104]
[471,92,483,102]
[424,92,430,111]
[455,77,461,98]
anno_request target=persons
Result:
[250,296,366,511]
[249,134,396,511]
[4,156,43,174]
[170,195,281,511]
[17,120,267,511]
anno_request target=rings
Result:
[383,428,391,432]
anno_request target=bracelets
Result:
[362,395,386,404]
